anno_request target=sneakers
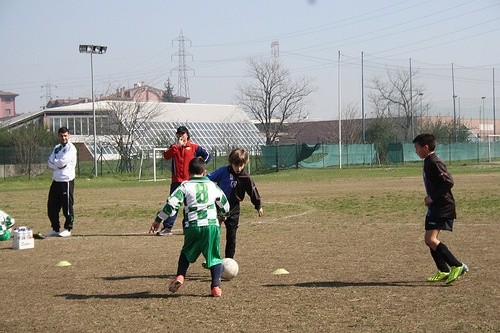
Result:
[444,262,469,284]
[44,230,60,237]
[58,229,72,237]
[426,270,451,283]
[156,226,174,236]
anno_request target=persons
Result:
[149,157,231,297]
[202,148,263,269]
[413,133,469,284]
[45,126,78,237]
[156,126,212,235]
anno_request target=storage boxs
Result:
[12,230,35,249]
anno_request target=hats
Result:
[175,125,189,133]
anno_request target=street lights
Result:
[453,95,458,134]
[78,44,108,176]
[419,92,424,131]
[480,96,486,140]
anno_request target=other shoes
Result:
[168,275,184,292]
[201,260,211,268]
[211,286,223,297]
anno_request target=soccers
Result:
[221,258,239,280]
